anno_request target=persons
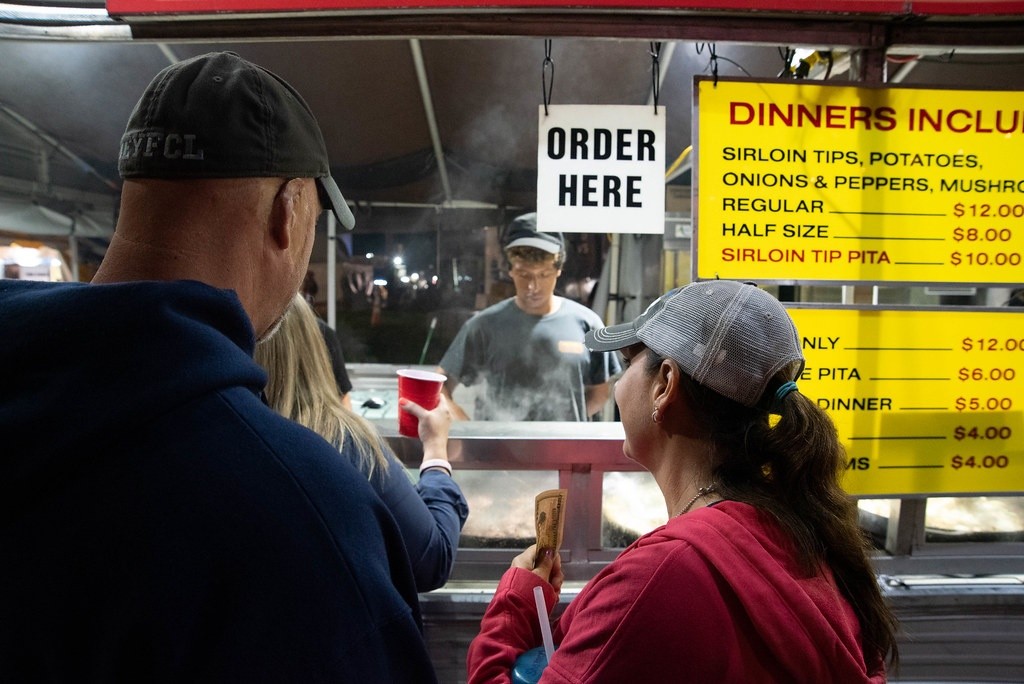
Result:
[467,281,903,684]
[299,266,352,409]
[0,53,433,684]
[251,294,469,594]
[439,212,621,424]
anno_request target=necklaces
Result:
[674,482,718,518]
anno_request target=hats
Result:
[116,51,358,229]
[584,279,806,406]
[501,212,565,255]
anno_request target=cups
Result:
[396,369,447,437]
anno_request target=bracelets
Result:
[419,459,452,477]
